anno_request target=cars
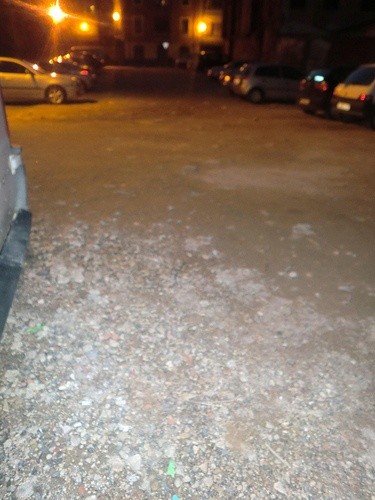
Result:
[33,50,104,103]
[208,58,303,103]
[297,65,355,119]
[0,56,86,104]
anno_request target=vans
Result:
[331,63,375,118]
